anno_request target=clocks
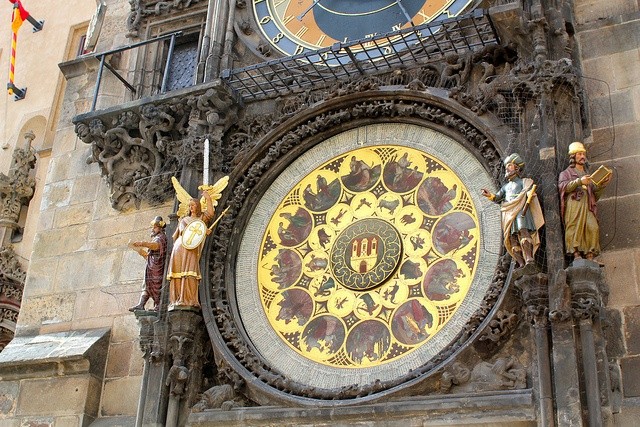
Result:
[251,0,482,67]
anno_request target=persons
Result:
[423,304,433,329]
[316,174,333,200]
[303,184,322,210]
[278,222,300,243]
[425,260,464,301]
[277,299,294,307]
[269,253,293,283]
[306,320,326,352]
[393,152,412,186]
[437,184,457,212]
[557,141,611,258]
[275,301,304,321]
[405,166,418,191]
[128,216,168,312]
[480,152,545,268]
[325,319,337,347]
[349,155,362,184]
[279,212,310,227]
[166,187,215,307]
[436,225,469,245]
